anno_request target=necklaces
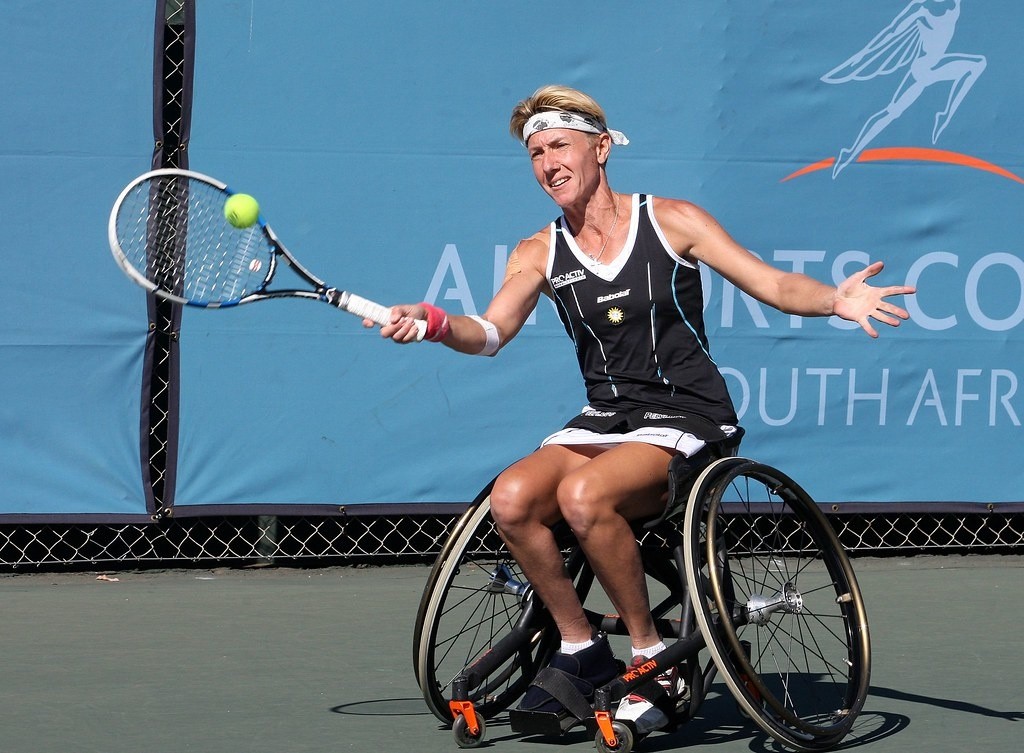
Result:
[564,191,620,265]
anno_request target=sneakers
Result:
[613,655,685,733]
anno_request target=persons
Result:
[361,83,919,738]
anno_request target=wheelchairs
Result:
[413,427,872,753]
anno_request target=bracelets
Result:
[417,303,449,343]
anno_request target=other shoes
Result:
[519,635,626,715]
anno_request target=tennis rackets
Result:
[108,167,428,342]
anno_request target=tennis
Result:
[223,193,258,228]
